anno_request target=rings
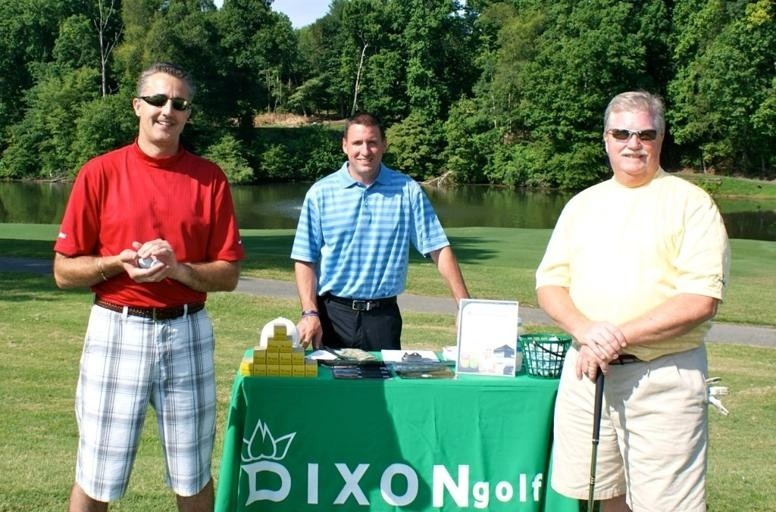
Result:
[164,247,171,258]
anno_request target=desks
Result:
[225,348,580,512]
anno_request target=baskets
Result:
[518,334,572,377]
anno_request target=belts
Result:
[609,354,646,365]
[94,297,204,320]
[326,290,398,312]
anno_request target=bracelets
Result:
[94,255,108,284]
[302,309,320,317]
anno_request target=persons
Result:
[53,64,246,512]
[535,90,732,512]
[290,111,472,351]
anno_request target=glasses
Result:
[139,95,190,110]
[606,129,659,141]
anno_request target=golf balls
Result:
[138,255,156,268]
